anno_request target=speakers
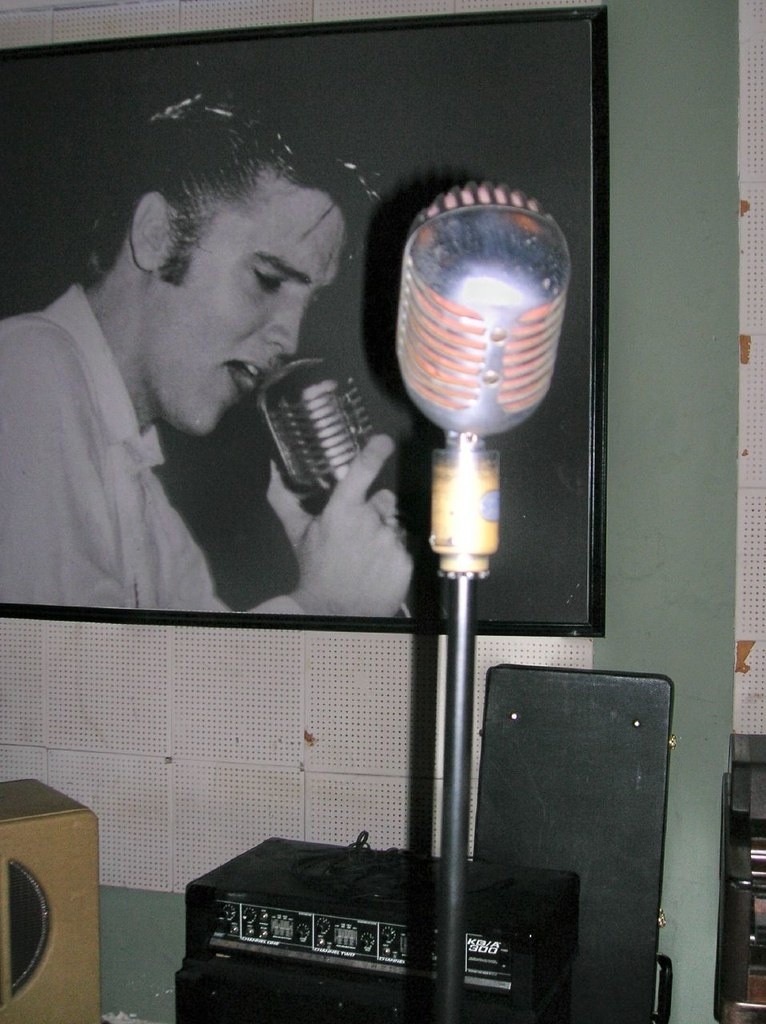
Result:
[0,779,103,1024]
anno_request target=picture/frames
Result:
[0,6,608,640]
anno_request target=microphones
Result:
[255,354,373,499]
[397,181,572,575]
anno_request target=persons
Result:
[0,97,416,618]
